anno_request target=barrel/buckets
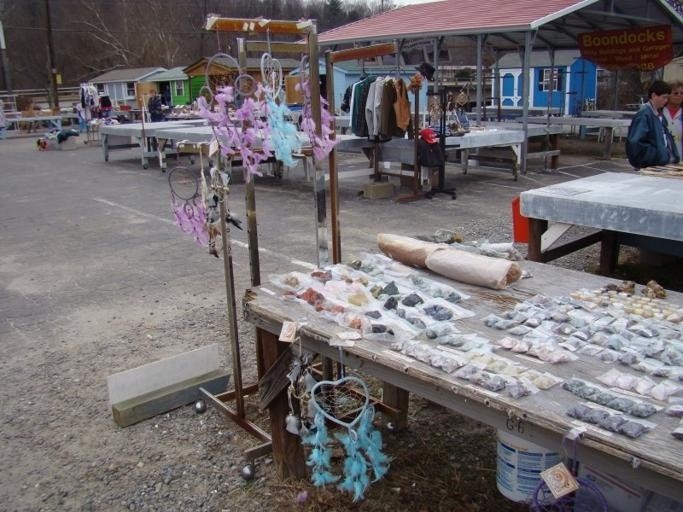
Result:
[512,197,547,243]
[496,428,569,504]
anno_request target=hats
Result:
[414,62,436,82]
[421,129,439,144]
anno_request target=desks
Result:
[244,161,682,507]
[99,111,638,189]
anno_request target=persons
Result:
[147,88,163,123]
[662,81,682,163]
[163,85,171,107]
[624,80,679,169]
